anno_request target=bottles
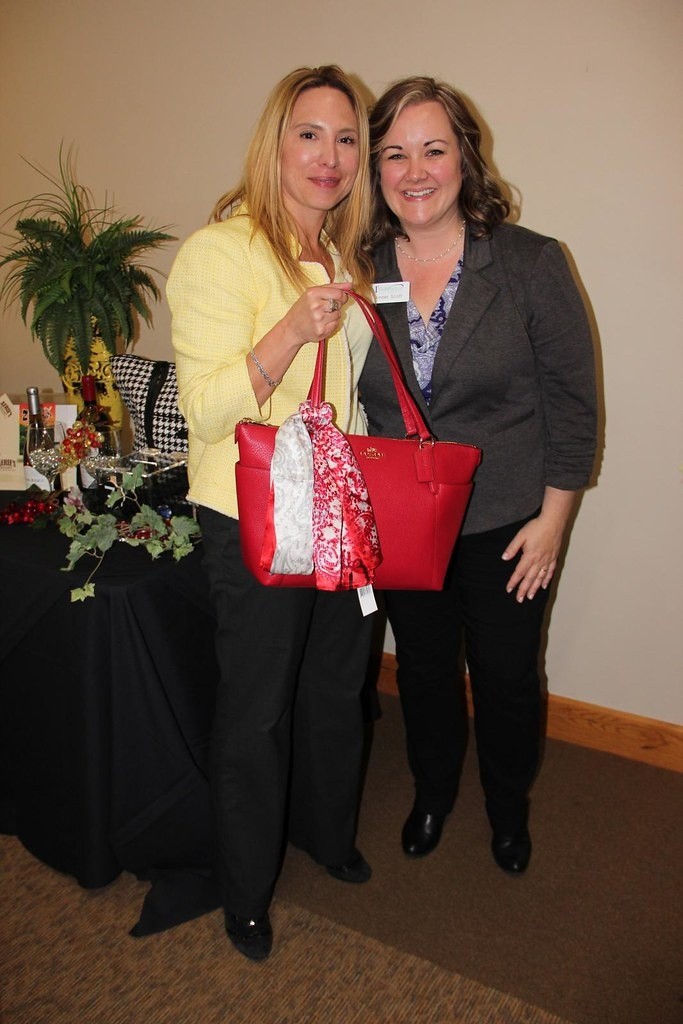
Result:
[23,387,62,492]
[77,376,113,493]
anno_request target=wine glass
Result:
[27,421,66,493]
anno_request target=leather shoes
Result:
[486,803,532,874]
[402,805,447,857]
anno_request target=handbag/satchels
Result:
[109,354,189,453]
[234,289,481,591]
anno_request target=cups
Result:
[82,425,120,485]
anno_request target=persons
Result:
[163,62,379,960]
[350,74,600,878]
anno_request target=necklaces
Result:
[394,217,465,262]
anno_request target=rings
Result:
[327,298,338,313]
[540,568,548,572]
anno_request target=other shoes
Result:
[225,909,272,959]
[327,846,373,883]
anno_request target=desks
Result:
[0,491,218,889]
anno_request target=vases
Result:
[60,315,123,452]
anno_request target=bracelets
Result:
[250,348,283,387]
